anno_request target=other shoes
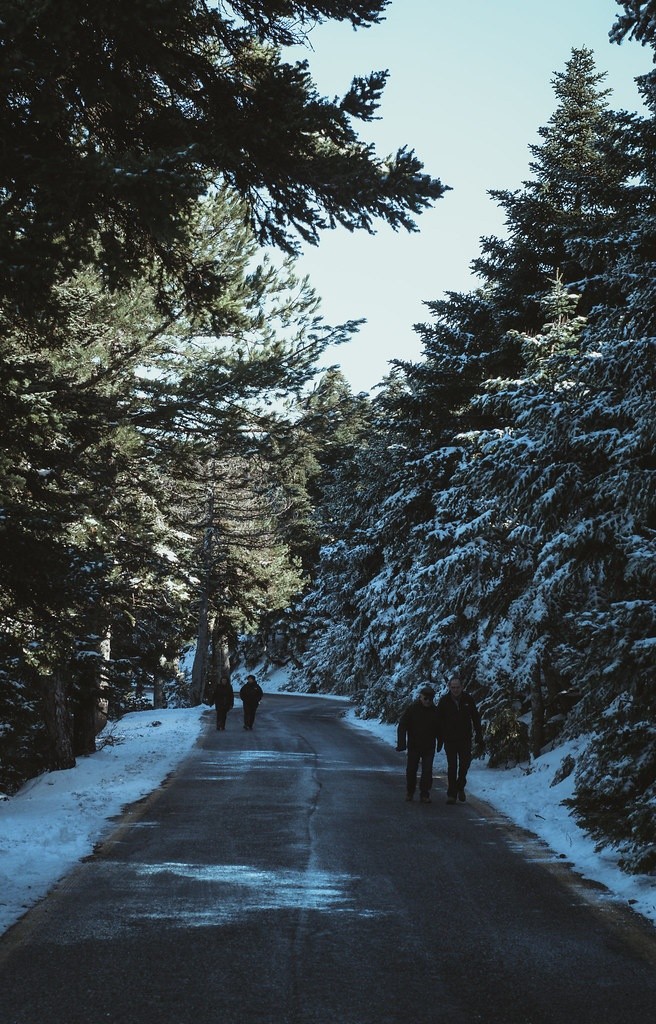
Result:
[457,787,466,802]
[216,727,225,731]
[447,797,456,804]
[406,790,414,801]
[243,725,252,730]
[421,796,431,803]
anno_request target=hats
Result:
[420,688,436,695]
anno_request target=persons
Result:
[437,677,482,804]
[211,677,234,730]
[398,688,443,804]
[240,675,264,730]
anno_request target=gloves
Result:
[396,744,406,751]
[475,727,482,740]
[437,736,444,752]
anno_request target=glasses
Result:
[424,698,431,701]
